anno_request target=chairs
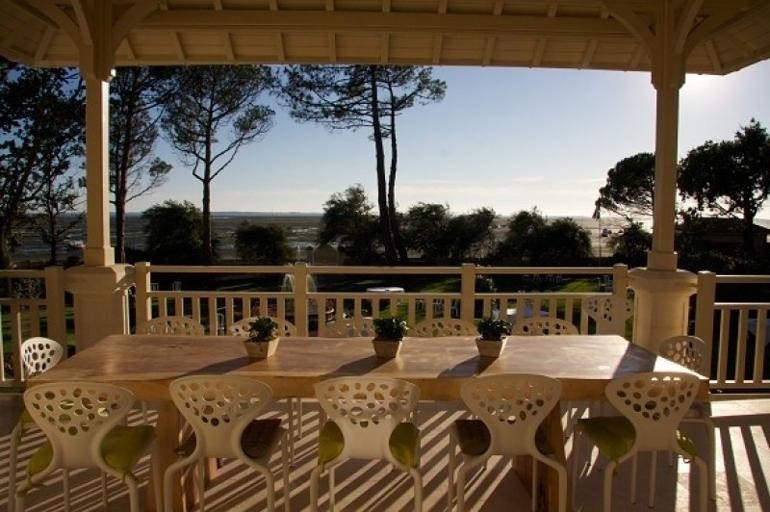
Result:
[514,316,578,335]
[314,317,378,424]
[584,293,633,335]
[310,377,424,512]
[571,369,708,512]
[9,336,112,511]
[411,317,479,425]
[138,317,204,424]
[629,336,712,505]
[228,316,304,467]
[16,382,162,512]
[163,376,292,512]
[449,374,568,512]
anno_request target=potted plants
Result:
[474,317,513,359]
[371,315,409,358]
[243,317,280,359]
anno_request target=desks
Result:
[26,335,709,512]
